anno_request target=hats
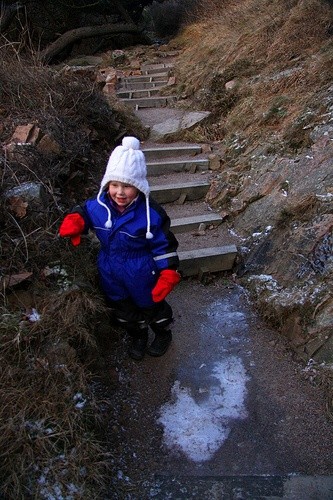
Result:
[101,136,150,199]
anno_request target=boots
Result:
[148,324,172,357]
[123,324,147,359]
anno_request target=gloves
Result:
[152,269,182,302]
[60,214,85,246]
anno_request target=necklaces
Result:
[59,135,181,357]
